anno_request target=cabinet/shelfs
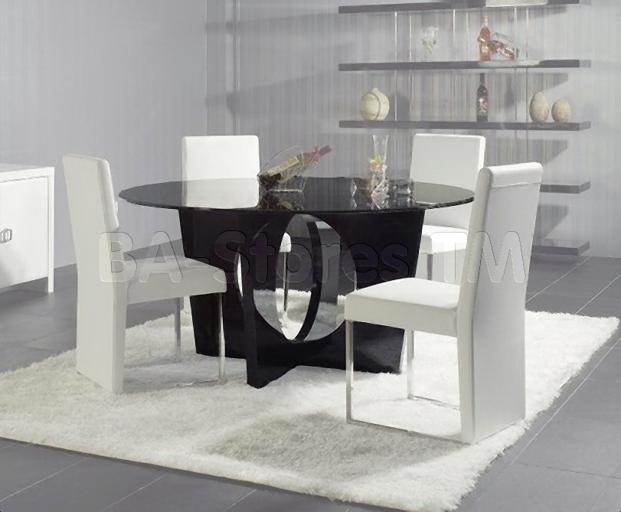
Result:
[0,161,56,296]
[333,3,589,256]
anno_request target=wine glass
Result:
[372,133,390,199]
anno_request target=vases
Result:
[359,87,390,121]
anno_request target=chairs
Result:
[62,131,546,447]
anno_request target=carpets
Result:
[0,297,621,511]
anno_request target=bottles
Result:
[478,15,491,61]
[474,72,489,124]
[477,35,519,62]
[258,144,333,192]
